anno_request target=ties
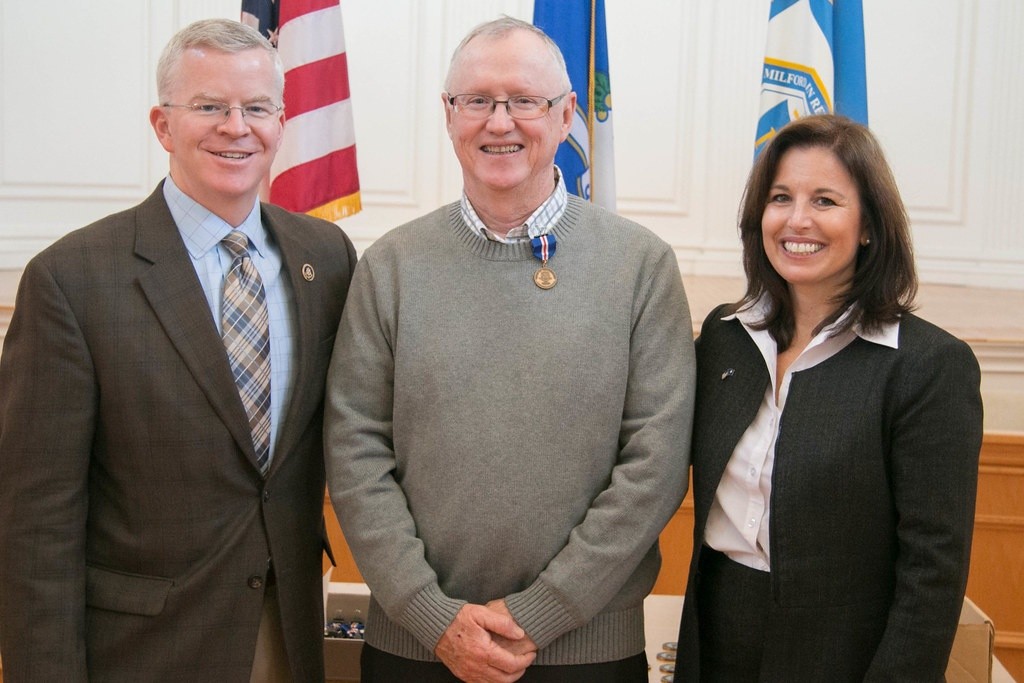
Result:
[220,230,272,469]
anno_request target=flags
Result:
[524,0,620,220]
[748,2,871,168]
[220,2,364,235]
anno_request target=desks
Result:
[324,565,1018,683]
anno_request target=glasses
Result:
[446,90,568,120]
[162,99,285,127]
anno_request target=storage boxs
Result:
[945,596,995,683]
[324,581,371,682]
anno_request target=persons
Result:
[318,13,697,683]
[3,14,358,683]
[678,116,987,683]
